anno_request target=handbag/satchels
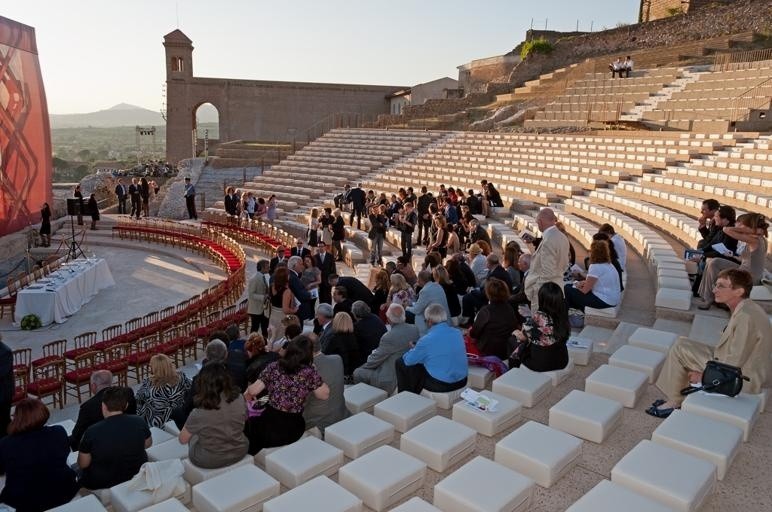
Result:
[701,360,742,397]
[263,297,270,318]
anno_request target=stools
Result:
[523,60,772,131]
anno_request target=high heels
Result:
[698,296,720,309]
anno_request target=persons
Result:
[74,182,85,226]
[690,205,738,298]
[644,268,771,420]
[0,338,13,478]
[609,57,623,78]
[1,399,79,510]
[39,202,52,247]
[184,177,197,220]
[697,199,719,247]
[75,388,152,496]
[88,192,100,230]
[619,55,634,78]
[246,179,626,394]
[136,314,330,468]
[224,185,277,224]
[116,176,159,220]
[70,369,137,450]
[697,213,769,310]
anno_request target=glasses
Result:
[711,282,739,289]
[296,242,303,244]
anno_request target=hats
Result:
[465,243,483,254]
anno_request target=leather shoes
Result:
[646,407,680,417]
[652,400,666,406]
